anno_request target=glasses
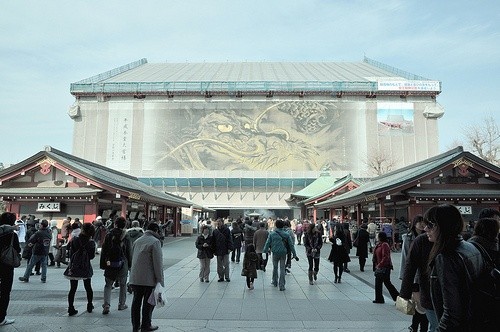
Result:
[424,220,437,229]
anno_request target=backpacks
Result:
[104,231,125,261]
[71,237,91,275]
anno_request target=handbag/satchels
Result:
[147,283,167,307]
[395,296,415,315]
[283,239,290,251]
[353,231,360,247]
[1,234,20,267]
[207,247,214,259]
[375,267,386,274]
[470,241,500,322]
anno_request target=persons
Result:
[262,220,298,291]
[63,223,95,316]
[129,222,164,332]
[0,204,500,332]
[19,220,51,283]
[241,244,259,290]
[196,226,214,282]
[373,232,400,303]
[0,212,21,326]
[100,217,131,314]
[212,218,233,282]
[303,224,323,285]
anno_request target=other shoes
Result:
[36,271,41,275]
[344,267,350,272]
[19,277,28,282]
[118,304,127,310]
[69,310,78,316]
[41,279,46,283]
[0,319,15,325]
[103,306,109,314]
[49,263,55,266]
[199,259,342,283]
[141,325,158,332]
[409,325,417,332]
[133,326,140,332]
[373,300,384,303]
[87,307,92,312]
[127,284,132,294]
